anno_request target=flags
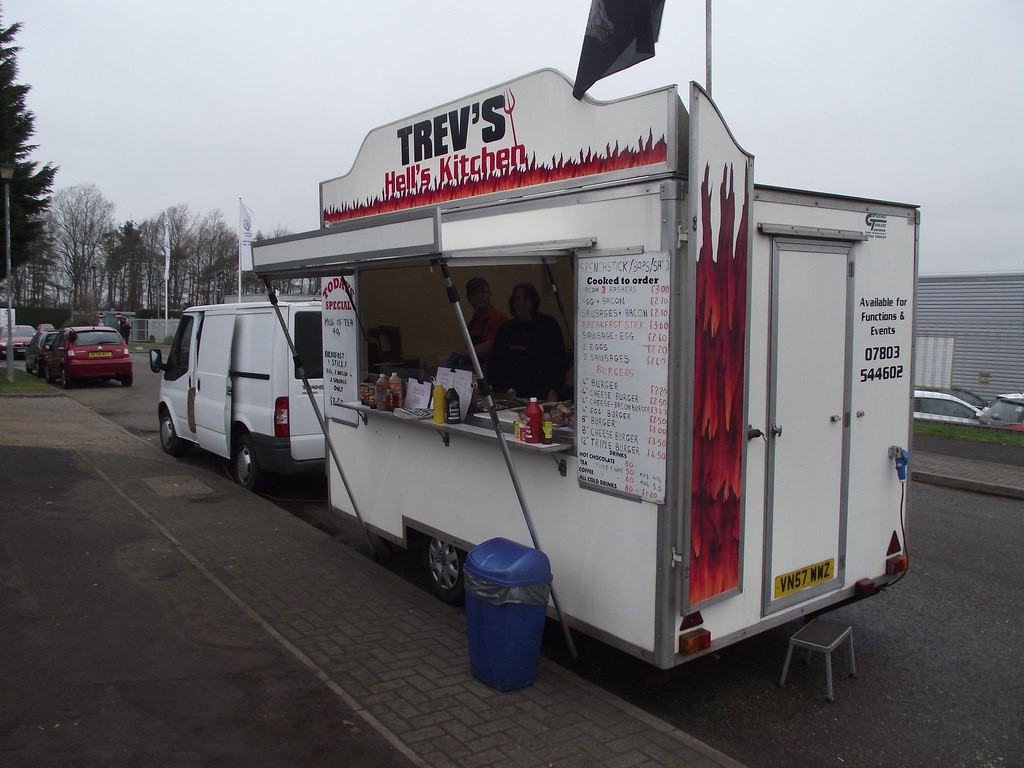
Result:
[573,0,666,100]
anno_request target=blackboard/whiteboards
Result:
[573,251,670,507]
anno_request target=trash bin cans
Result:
[461,537,553,693]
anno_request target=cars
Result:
[0,323,133,390]
[913,388,1024,431]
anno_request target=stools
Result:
[780,618,858,702]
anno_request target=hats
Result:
[466,277,487,295]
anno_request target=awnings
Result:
[251,207,594,656]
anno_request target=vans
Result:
[149,300,325,492]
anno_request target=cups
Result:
[514,419,526,442]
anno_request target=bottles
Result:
[433,382,447,424]
[386,389,396,412]
[525,397,543,443]
[389,373,403,408]
[445,385,461,424]
[542,406,553,444]
[376,373,389,411]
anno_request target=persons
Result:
[122,318,131,344]
[490,283,566,400]
[459,278,507,380]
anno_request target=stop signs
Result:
[98,313,104,319]
[116,313,122,319]
